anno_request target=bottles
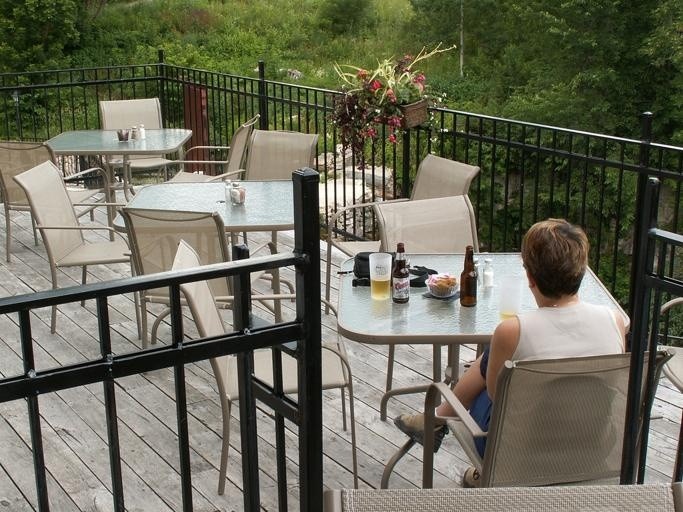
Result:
[131,126,138,139]
[460,246,478,307]
[224,180,231,197]
[393,243,410,303]
[483,259,494,286]
[139,124,145,138]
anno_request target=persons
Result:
[394,218,625,488]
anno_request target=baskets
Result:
[400,99,428,130]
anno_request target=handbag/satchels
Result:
[353,251,437,288]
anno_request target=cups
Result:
[369,253,392,301]
[499,275,520,320]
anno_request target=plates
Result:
[426,280,460,298]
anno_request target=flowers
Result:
[324,40,457,170]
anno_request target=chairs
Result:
[651,295,683,437]
[170,242,359,494]
[0,142,100,261]
[100,98,167,192]
[245,128,320,180]
[324,153,481,314]
[120,206,279,340]
[421,348,675,489]
[320,482,683,512]
[374,195,479,394]
[156,113,260,182]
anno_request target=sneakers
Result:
[394,411,448,455]
[464,466,479,486]
